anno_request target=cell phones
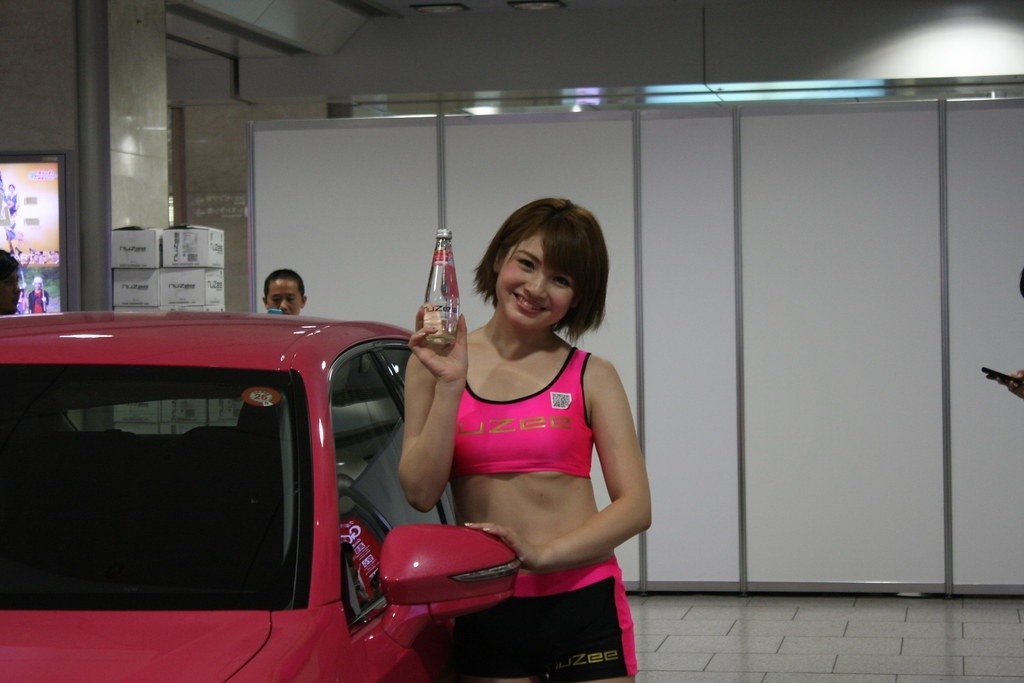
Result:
[268,308,283,314]
[982,367,1023,384]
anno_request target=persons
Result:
[398,198,652,683]
[29,277,49,313]
[6,184,21,253]
[262,269,307,315]
[982,266,1024,399]
[0,250,22,315]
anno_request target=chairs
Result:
[156,424,283,587]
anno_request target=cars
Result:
[0,307,521,683]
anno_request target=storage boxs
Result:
[110,224,226,311]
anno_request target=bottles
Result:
[422,227,461,345]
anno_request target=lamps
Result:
[409,2,469,15]
[504,0,568,12]
